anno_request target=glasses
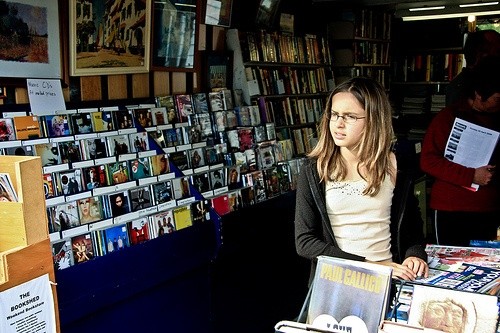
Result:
[326,111,370,123]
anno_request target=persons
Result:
[161,217,172,235]
[108,237,114,253]
[118,235,123,250]
[113,165,127,183]
[122,234,127,248]
[79,198,101,225]
[230,169,238,185]
[88,139,102,159]
[167,217,174,232]
[158,220,163,237]
[72,180,79,193]
[52,116,64,136]
[420,69,500,247]
[213,170,222,189]
[140,137,145,151]
[113,195,129,217]
[218,146,225,162]
[295,76,429,282]
[67,177,74,195]
[113,237,118,251]
[87,168,100,190]
[135,136,142,152]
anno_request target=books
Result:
[0,87,293,273]
[274,238,500,333]
[239,8,464,176]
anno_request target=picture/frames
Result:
[68,0,202,76]
[0,0,64,80]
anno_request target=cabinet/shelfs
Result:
[229,0,467,162]
